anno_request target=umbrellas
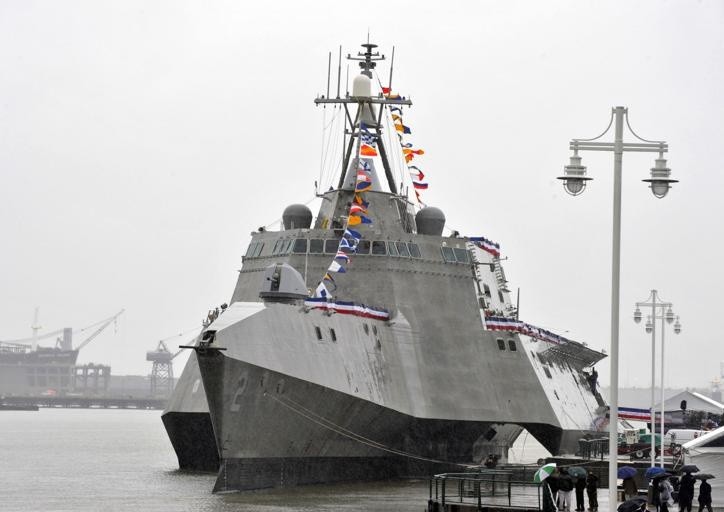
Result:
[679,465,699,473]
[617,466,638,479]
[617,499,648,512]
[645,466,667,477]
[534,463,557,483]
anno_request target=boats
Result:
[162,41,604,495]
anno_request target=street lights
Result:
[557,108,681,512]
[634,290,682,472]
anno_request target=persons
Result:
[636,504,649,512]
[543,467,599,512]
[650,472,714,512]
[622,477,638,501]
